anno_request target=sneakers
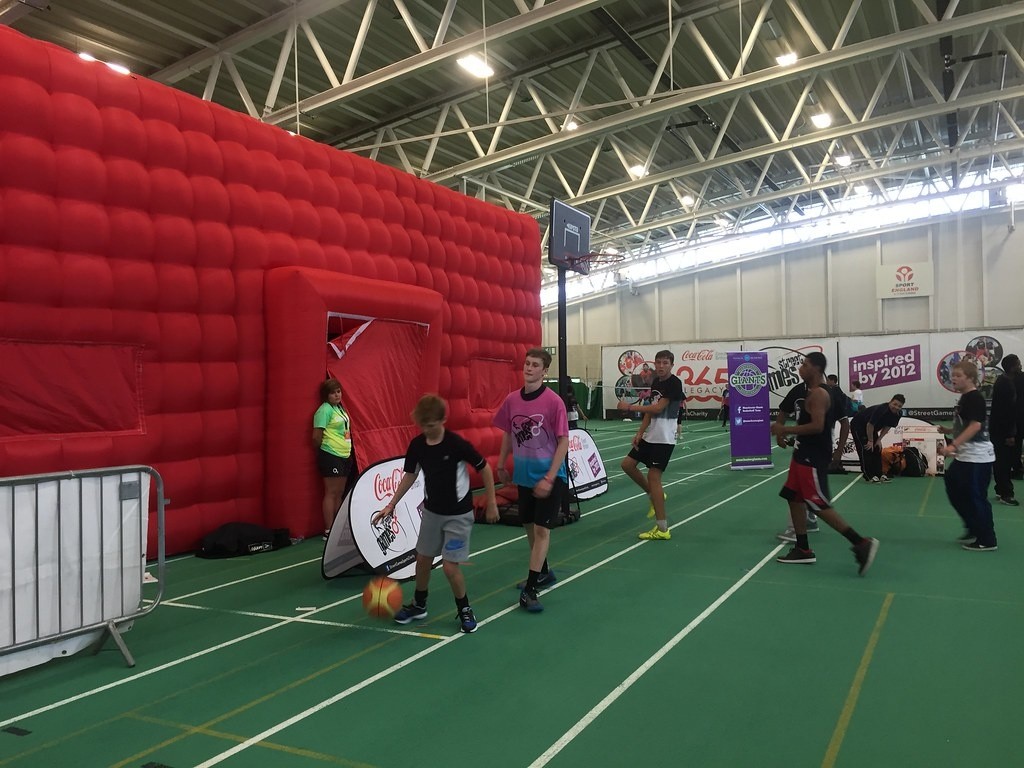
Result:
[853,536,879,576]
[639,524,671,540]
[776,546,817,564]
[519,587,544,611]
[962,541,998,551]
[805,518,820,533]
[778,530,799,542]
[996,492,1019,506]
[647,492,666,519]
[455,607,477,633]
[394,598,429,624]
[516,570,557,591]
[959,533,977,544]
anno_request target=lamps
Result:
[799,90,832,129]
[823,139,851,168]
[457,53,694,207]
[762,17,797,66]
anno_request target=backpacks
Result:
[901,446,928,476]
[844,393,858,415]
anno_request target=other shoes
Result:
[870,477,881,485]
[322,533,329,541]
[880,476,892,482]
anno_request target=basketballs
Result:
[362,577,402,617]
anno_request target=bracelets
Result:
[544,476,555,484]
[497,467,504,471]
[386,502,395,511]
[628,405,631,411]
[951,443,958,450]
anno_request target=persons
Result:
[566,385,589,429]
[492,346,569,611]
[677,392,690,439]
[721,384,729,428]
[827,375,842,410]
[312,378,351,540]
[639,383,651,405]
[372,395,500,633]
[937,361,998,551]
[643,397,650,405]
[776,382,849,541]
[771,352,880,575]
[850,394,905,484]
[851,381,863,403]
[988,353,1024,506]
[617,348,682,540]
